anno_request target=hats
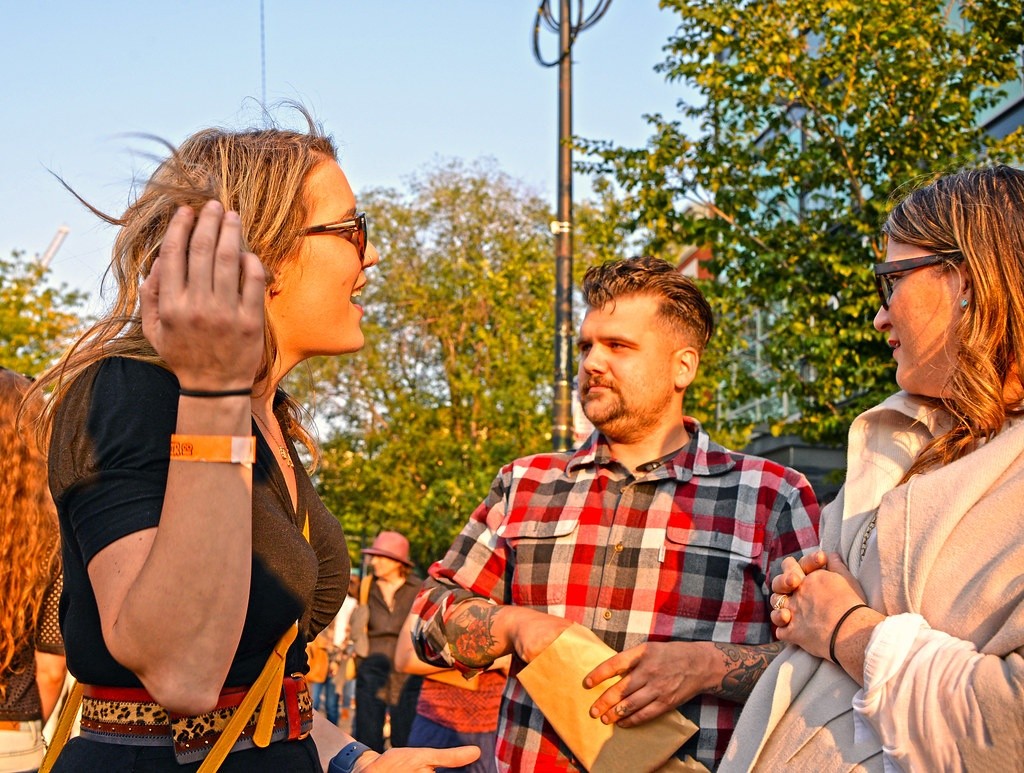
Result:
[360,531,416,568]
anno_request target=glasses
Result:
[299,211,368,258]
[874,252,964,311]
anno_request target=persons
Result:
[1,101,1024,770]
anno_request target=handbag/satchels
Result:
[346,573,374,659]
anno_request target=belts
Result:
[76,671,315,766]
